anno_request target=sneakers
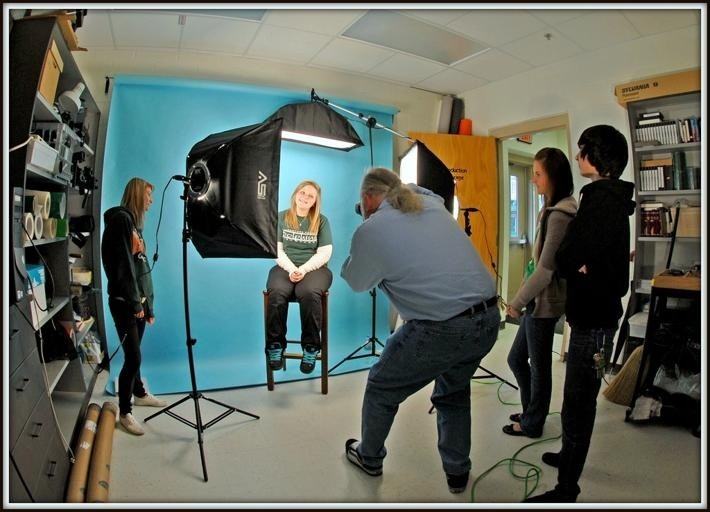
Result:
[300,345,320,374]
[448,473,470,493]
[119,411,145,437]
[266,338,288,371]
[132,394,168,407]
[345,438,385,476]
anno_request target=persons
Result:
[339,168,500,492]
[522,124,636,502]
[502,147,577,438]
[266,180,334,372]
[102,178,168,436]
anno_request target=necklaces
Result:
[297,216,306,228]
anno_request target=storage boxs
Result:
[10,17,104,457]
[623,89,701,384]
[614,68,701,109]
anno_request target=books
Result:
[634,111,700,144]
[641,201,665,237]
[640,158,673,191]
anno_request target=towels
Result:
[629,394,662,419]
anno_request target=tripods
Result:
[143,191,261,483]
[427,218,519,414]
[326,98,416,374]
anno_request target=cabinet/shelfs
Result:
[9,294,72,502]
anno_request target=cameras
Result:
[354,203,363,216]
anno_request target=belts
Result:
[463,296,497,315]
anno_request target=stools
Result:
[263,290,329,395]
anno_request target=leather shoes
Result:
[503,423,527,437]
[510,411,523,421]
[542,450,567,470]
[522,490,577,503]
